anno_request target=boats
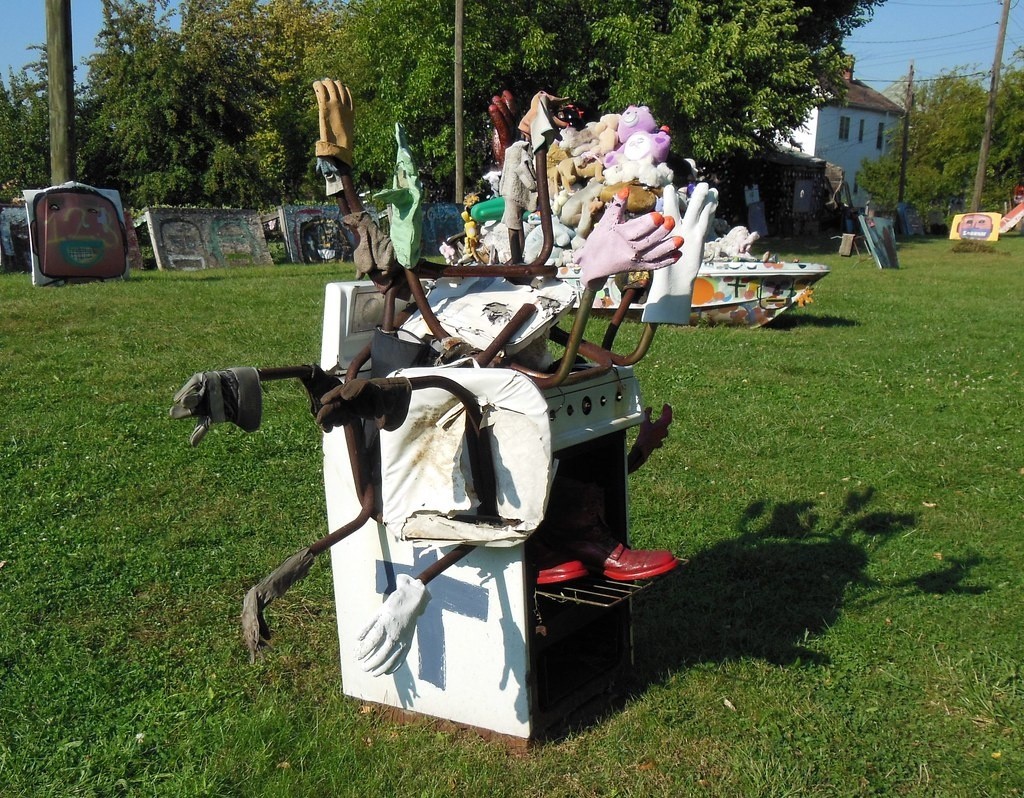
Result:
[556,251,831,329]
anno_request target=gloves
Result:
[316,155,344,197]
[311,76,356,169]
[575,183,686,286]
[169,367,264,447]
[499,141,537,233]
[372,118,422,270]
[359,327,427,450]
[354,573,431,677]
[639,183,720,327]
[316,376,412,432]
[300,362,340,416]
[516,89,574,155]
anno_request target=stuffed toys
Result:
[522,106,698,263]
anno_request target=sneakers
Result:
[543,517,677,581]
[525,539,588,587]
